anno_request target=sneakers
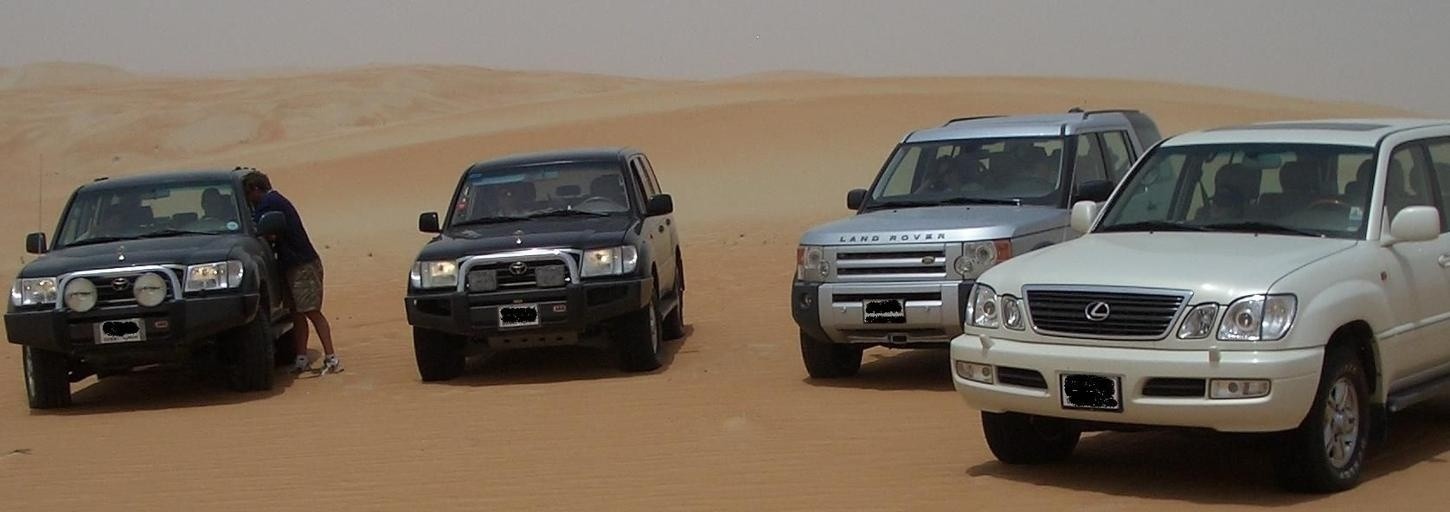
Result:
[319,356,344,374]
[284,358,311,375]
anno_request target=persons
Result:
[200,187,224,219]
[493,184,529,217]
[1209,183,1244,220]
[241,169,342,377]
[913,154,983,196]
[74,203,136,242]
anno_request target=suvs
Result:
[790,108,1161,378]
[949,119,1450,492]
[5,166,295,408]
[405,146,685,381]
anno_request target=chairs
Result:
[514,173,628,215]
[1194,156,1449,238]
[955,148,1117,197]
[140,195,233,231]
